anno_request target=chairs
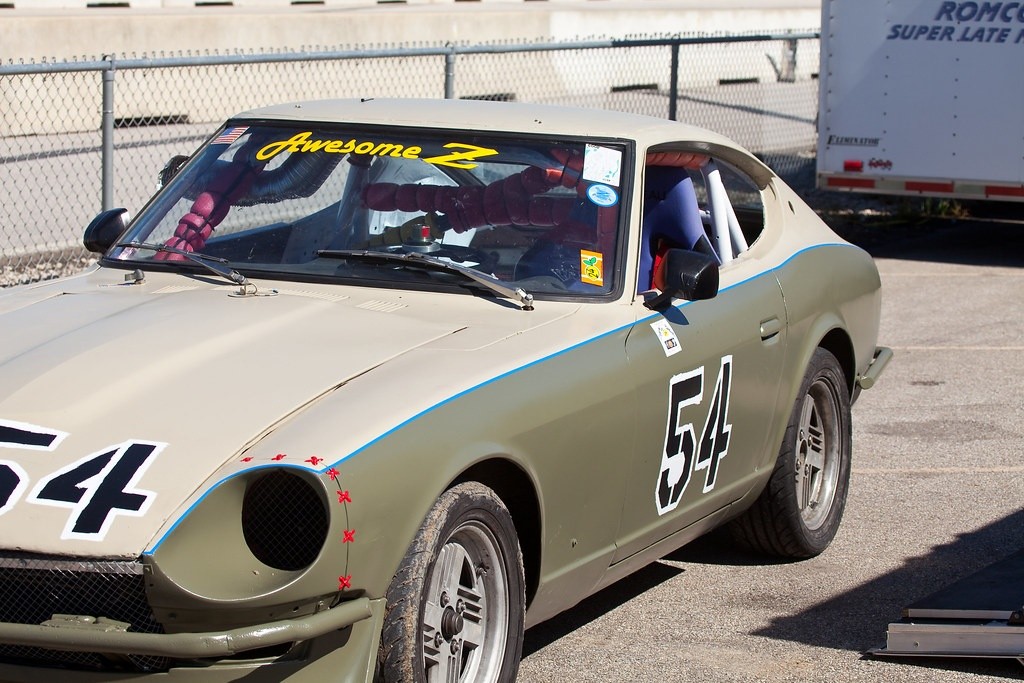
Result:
[514,165,720,290]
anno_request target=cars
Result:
[0,95,892,682]
